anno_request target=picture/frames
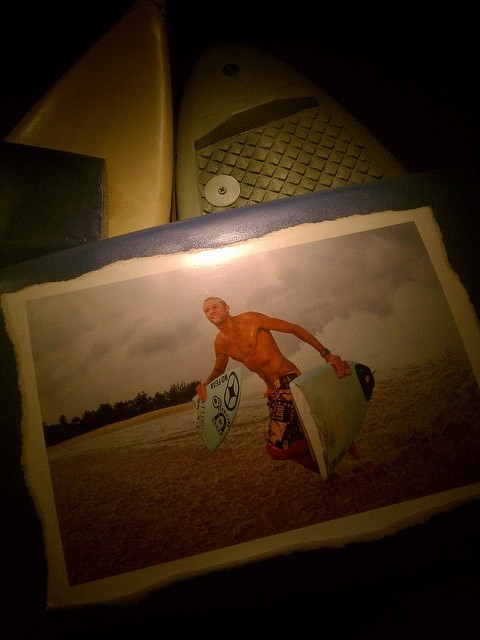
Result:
[0,175,480,606]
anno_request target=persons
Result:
[195,296,362,481]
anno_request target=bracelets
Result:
[320,348,330,357]
